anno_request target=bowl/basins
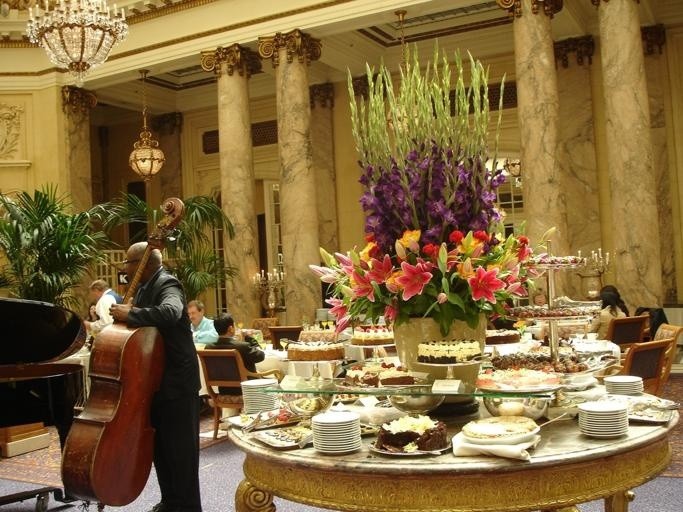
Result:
[385,385,444,416]
[481,395,549,422]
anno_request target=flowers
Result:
[308,35,557,334]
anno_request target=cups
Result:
[357,329,393,339]
[569,330,597,343]
[301,319,333,331]
[288,340,330,348]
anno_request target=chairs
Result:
[604,313,681,399]
[194,348,286,440]
[604,315,650,352]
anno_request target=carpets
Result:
[631,376,681,477]
[0,409,227,493]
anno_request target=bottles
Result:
[266,341,273,356]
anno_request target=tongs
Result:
[241,410,277,435]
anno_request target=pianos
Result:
[0,297,89,498]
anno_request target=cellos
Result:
[61,198,187,507]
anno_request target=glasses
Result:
[123,259,139,266]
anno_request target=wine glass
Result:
[279,339,287,356]
[236,320,243,335]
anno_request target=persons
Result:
[600,286,620,300]
[188,300,220,414]
[83,279,124,337]
[203,313,272,396]
[84,303,100,353]
[588,291,627,341]
[533,288,548,307]
[109,242,202,512]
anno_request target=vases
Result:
[391,309,488,364]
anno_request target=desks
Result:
[269,297,621,357]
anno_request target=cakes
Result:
[287,309,592,389]
[462,415,539,441]
[374,413,450,454]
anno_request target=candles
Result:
[574,248,610,267]
[252,269,285,282]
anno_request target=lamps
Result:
[21,1,129,89]
[129,66,164,185]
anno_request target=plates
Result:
[309,412,362,453]
[603,375,644,395]
[369,442,451,456]
[315,309,336,321]
[461,427,541,446]
[576,402,629,438]
[240,378,279,413]
